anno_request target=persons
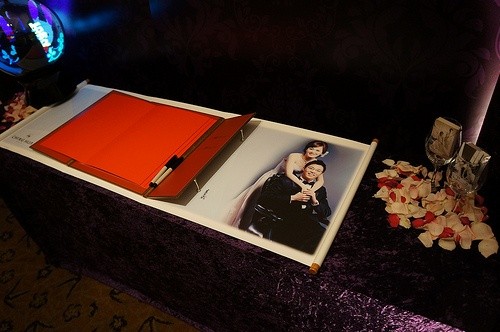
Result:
[444,128,455,154]
[464,161,475,182]
[434,131,445,153]
[228,140,329,230]
[454,161,464,176]
[272,159,331,254]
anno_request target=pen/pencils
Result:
[153,157,184,188]
[149,154,178,187]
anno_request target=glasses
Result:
[307,165,324,174]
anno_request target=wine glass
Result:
[426,117,463,186]
[446,145,491,213]
